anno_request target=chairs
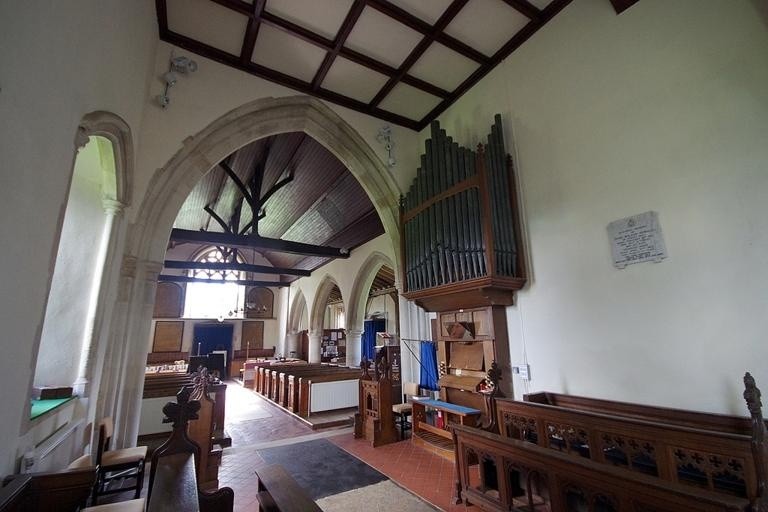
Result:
[137,371,195,437]
[92,416,148,505]
[392,383,418,440]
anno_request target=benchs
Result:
[254,362,381,418]
[409,399,480,463]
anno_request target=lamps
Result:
[157,57,199,112]
[377,126,397,168]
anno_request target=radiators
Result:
[19,417,86,476]
